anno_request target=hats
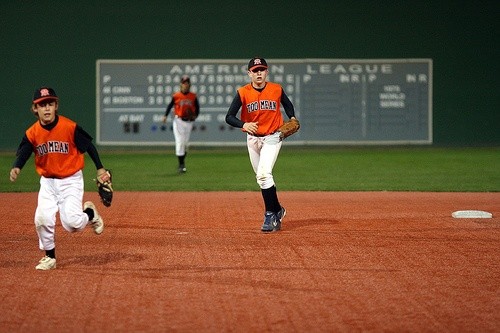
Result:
[248,57,268,72]
[33,87,59,104]
[181,77,190,84]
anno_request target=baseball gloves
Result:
[181,108,195,121]
[96,170,114,207]
[277,119,300,141]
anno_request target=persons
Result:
[225,57,301,231]
[162,75,199,172]
[10,89,113,271]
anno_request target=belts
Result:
[248,132,275,137]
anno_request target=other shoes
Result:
[178,166,187,172]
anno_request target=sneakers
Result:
[35,255,56,270]
[277,207,287,222]
[261,211,281,233]
[84,201,104,234]
